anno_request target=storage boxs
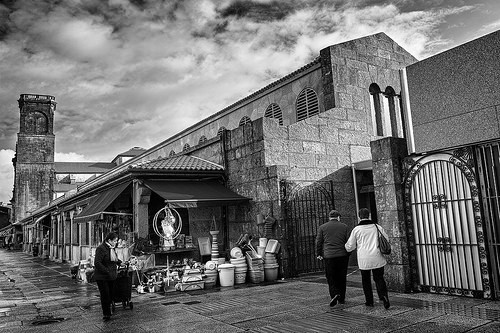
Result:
[180,269,205,291]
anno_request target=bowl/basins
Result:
[209,230,220,235]
[206,261,216,270]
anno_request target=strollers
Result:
[111,260,134,312]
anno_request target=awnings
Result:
[34,212,51,228]
[141,180,253,202]
[73,180,134,223]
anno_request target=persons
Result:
[314,209,352,306]
[5,236,9,250]
[345,208,391,309]
[94,232,126,322]
[42,234,50,252]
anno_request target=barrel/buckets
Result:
[259,238,267,247]
[264,254,279,282]
[217,247,247,287]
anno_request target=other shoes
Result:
[329,294,340,308]
[363,299,374,306]
[382,295,390,309]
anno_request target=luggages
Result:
[110,260,133,312]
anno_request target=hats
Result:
[329,210,342,217]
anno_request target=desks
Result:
[142,249,203,263]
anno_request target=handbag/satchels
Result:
[375,224,391,254]
